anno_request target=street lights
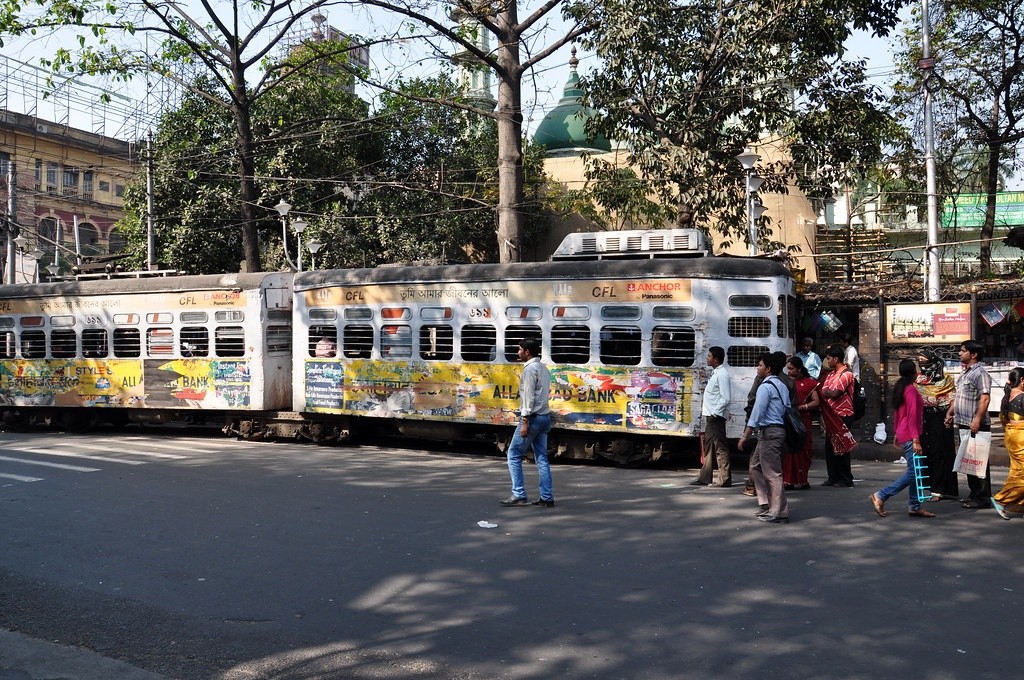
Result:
[735,145,769,256]
[273,196,321,273]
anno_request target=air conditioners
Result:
[36,124,48,133]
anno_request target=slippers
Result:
[908,509,935,518]
[990,497,1010,520]
[868,493,886,517]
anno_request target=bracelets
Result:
[913,442,920,444]
[801,404,804,410]
[805,404,808,410]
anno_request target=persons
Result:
[689,346,731,487]
[315,330,337,358]
[737,333,860,524]
[943,340,994,508]
[0,331,208,358]
[912,351,959,502]
[869,359,936,518]
[499,339,555,507]
[990,367,1024,520]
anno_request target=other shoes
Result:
[794,483,811,490]
[754,504,769,515]
[833,480,854,487]
[689,480,707,485]
[959,497,972,502]
[759,515,789,523]
[961,500,991,508]
[821,480,835,486]
[741,488,754,496]
[709,482,721,487]
[784,484,794,490]
[926,493,943,502]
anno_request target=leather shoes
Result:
[532,498,554,507]
[499,496,527,504]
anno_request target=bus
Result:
[0,211,802,466]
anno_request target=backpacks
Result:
[840,368,866,420]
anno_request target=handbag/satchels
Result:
[783,407,807,454]
[952,429,991,479]
